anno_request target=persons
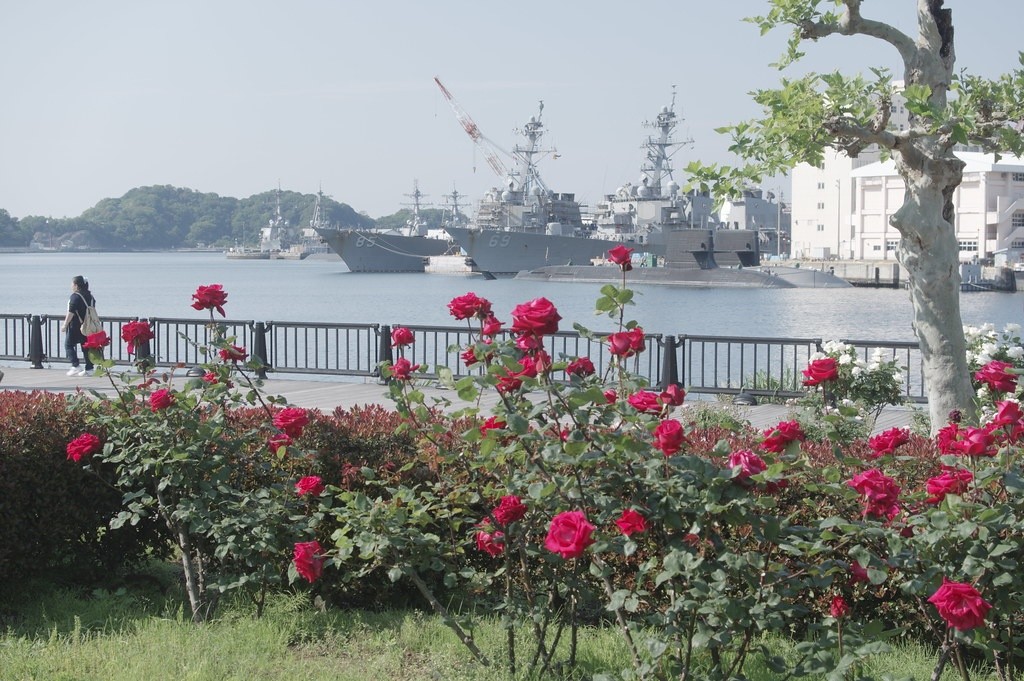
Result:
[60,275,97,378]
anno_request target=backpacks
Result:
[70,290,103,337]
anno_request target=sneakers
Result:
[66,363,84,377]
[79,368,95,378]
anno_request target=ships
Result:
[221,77,611,273]
[442,80,789,280]
